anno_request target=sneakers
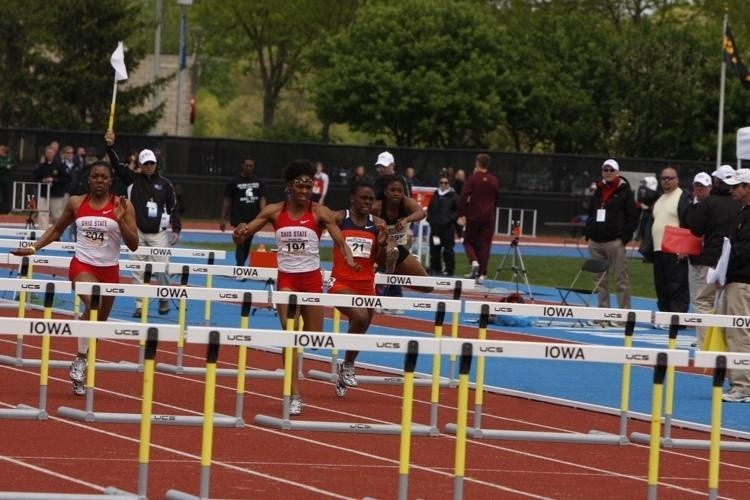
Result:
[721,386,749,403]
[335,363,349,398]
[478,273,488,280]
[68,355,86,383]
[341,360,358,387]
[132,308,142,318]
[159,300,170,314]
[470,260,480,279]
[72,381,86,395]
[289,397,304,415]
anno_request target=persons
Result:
[105,133,182,317]
[310,162,329,206]
[327,180,389,399]
[10,161,139,395]
[426,176,459,277]
[0,146,16,216]
[638,167,690,330]
[683,172,712,207]
[722,166,750,402]
[373,151,411,200]
[35,140,86,229]
[584,159,639,327]
[459,154,500,285]
[689,165,735,350]
[370,174,433,294]
[233,161,362,417]
[219,159,266,281]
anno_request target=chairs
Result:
[547,259,611,328]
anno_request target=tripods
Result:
[483,219,534,304]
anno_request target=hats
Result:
[138,148,157,165]
[711,164,735,181]
[601,158,619,171]
[374,151,395,168]
[722,167,750,186]
[693,171,712,187]
[80,146,98,156]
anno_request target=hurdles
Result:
[308,270,475,390]
[693,350,750,498]
[1,277,72,421]
[167,324,690,500]
[630,312,750,450]
[254,290,462,437]
[0,253,168,373]
[444,300,652,446]
[155,261,323,380]
[56,281,269,428]
[1,238,227,328]
[0,317,179,499]
[0,226,49,312]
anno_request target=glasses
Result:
[439,181,448,185]
[661,176,675,180]
[603,168,615,172]
